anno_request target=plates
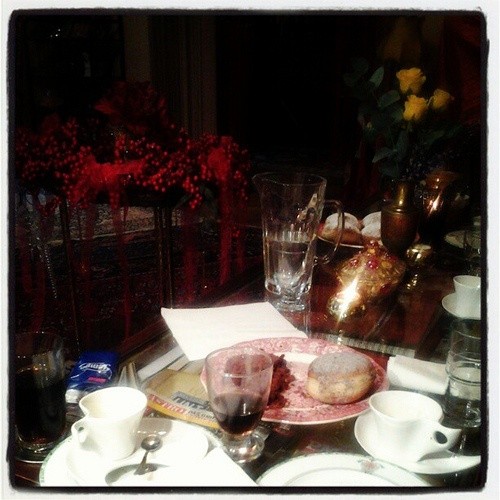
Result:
[354,413,481,475]
[256,450,431,487]
[314,221,365,251]
[38,419,223,486]
[199,337,386,427]
[441,294,481,322]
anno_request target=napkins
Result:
[160,300,309,361]
[386,354,482,400]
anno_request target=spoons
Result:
[133,435,162,476]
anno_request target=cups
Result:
[444,325,484,428]
[204,348,274,463]
[410,170,455,271]
[368,391,450,461]
[252,171,345,312]
[453,275,481,315]
[463,215,481,277]
[8,330,68,451]
[380,202,418,254]
[71,387,148,458]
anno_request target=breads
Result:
[321,211,381,245]
[305,351,375,404]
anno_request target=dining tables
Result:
[9,173,482,487]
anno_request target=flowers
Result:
[342,59,476,184]
